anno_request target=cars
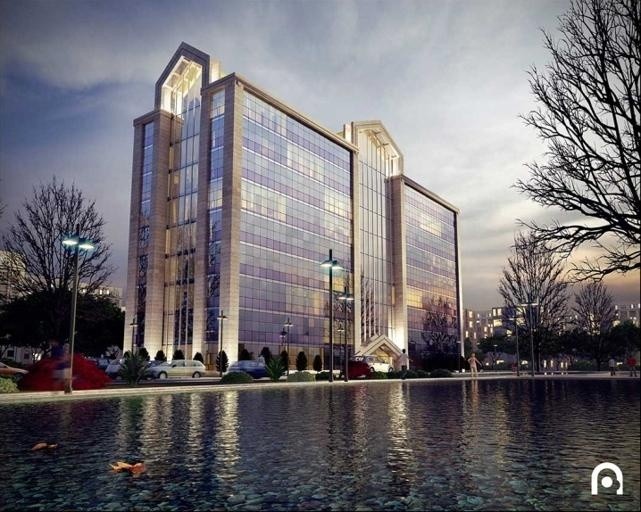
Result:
[0,362,28,382]
[350,355,393,373]
[228,360,270,379]
[145,359,207,379]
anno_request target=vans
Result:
[105,359,125,379]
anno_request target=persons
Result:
[608,357,617,376]
[53,343,76,385]
[400,349,414,378]
[468,352,482,376]
[627,354,639,377]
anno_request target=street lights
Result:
[337,324,345,372]
[204,310,227,377]
[337,286,355,382]
[509,303,539,377]
[62,224,95,395]
[280,318,294,376]
[129,319,139,354]
[320,249,344,382]
